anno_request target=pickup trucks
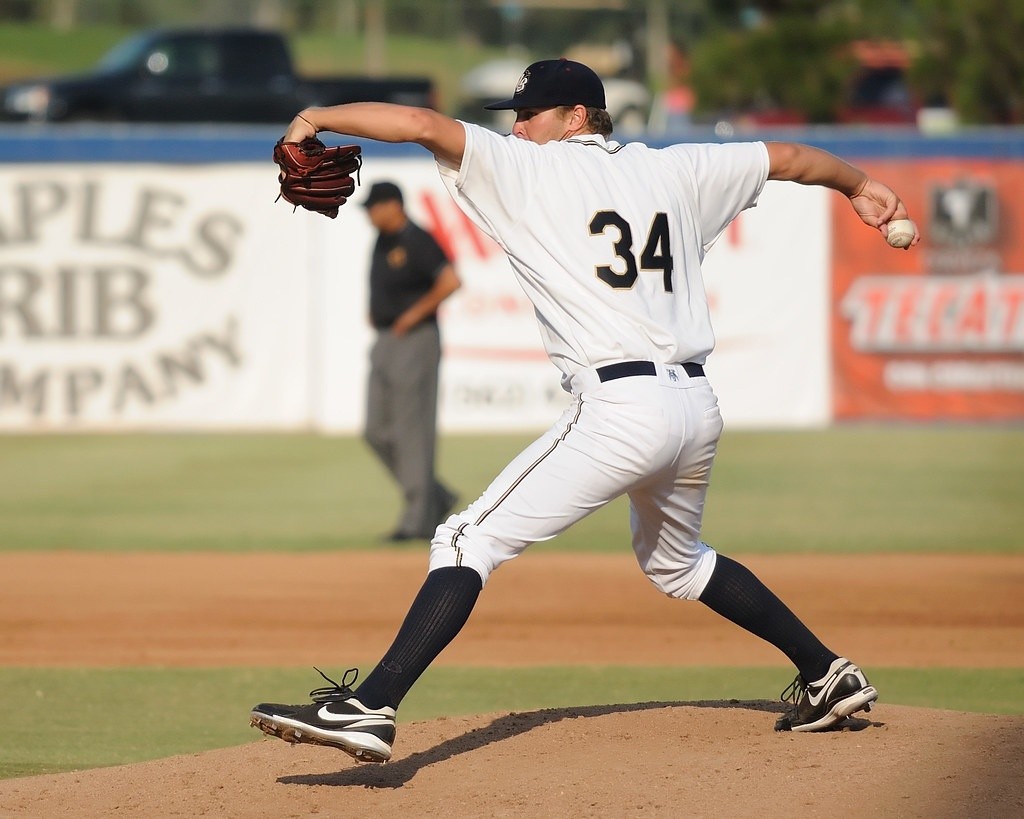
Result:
[0,25,436,133]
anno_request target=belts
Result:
[596,361,706,382]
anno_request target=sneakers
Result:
[774,657,878,731]
[250,666,396,763]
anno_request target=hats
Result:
[483,58,605,110]
[359,182,402,206]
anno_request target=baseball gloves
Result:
[271,136,365,219]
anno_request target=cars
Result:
[730,41,1024,126]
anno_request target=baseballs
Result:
[885,219,915,247]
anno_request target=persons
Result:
[249,57,921,765]
[352,180,463,548]
